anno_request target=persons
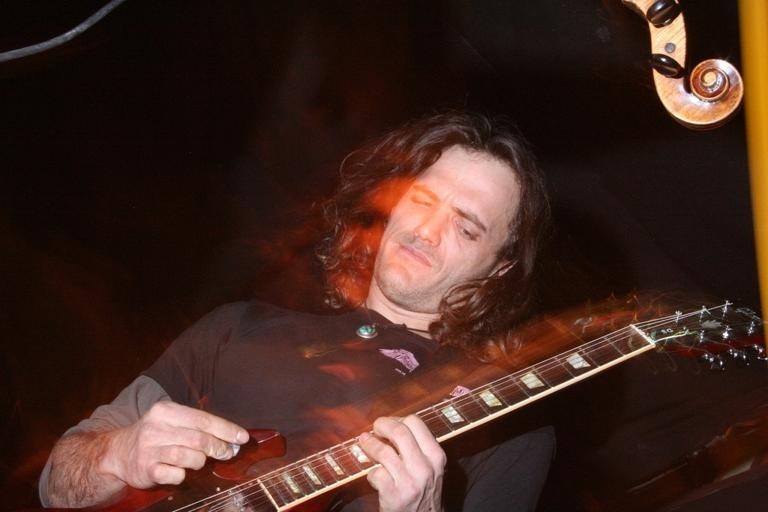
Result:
[38,111,557,511]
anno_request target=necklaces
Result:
[355,299,431,339]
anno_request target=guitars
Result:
[74,293,767,511]
